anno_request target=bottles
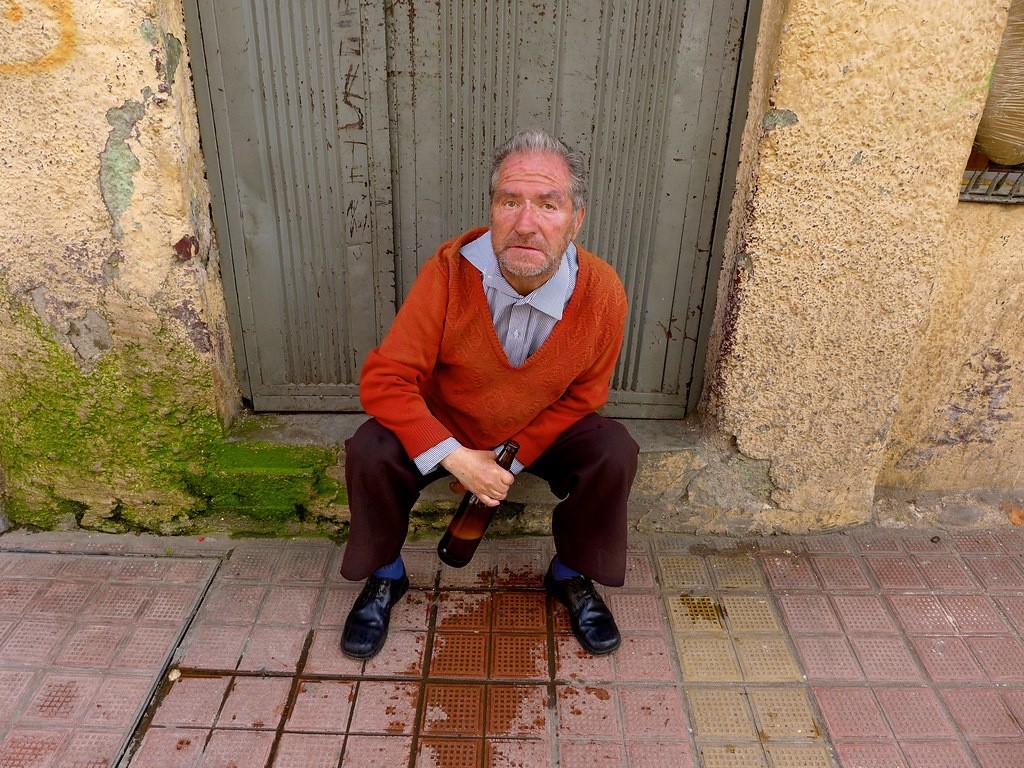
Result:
[437,439,520,568]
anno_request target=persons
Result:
[341,128,642,660]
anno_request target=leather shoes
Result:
[543,554,621,654]
[340,562,410,660]
